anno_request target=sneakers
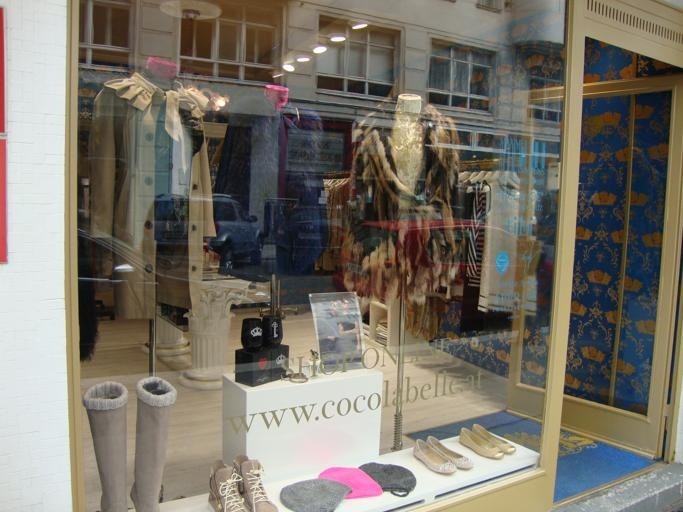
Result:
[208,459,251,512]
[460,427,505,459]
[232,455,278,512]
[471,423,516,454]
[426,436,473,469]
[413,439,457,473]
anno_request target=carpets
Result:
[405,408,665,504]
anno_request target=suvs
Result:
[143,192,265,264]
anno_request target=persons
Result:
[321,321,358,354]
[213,84,329,282]
[338,91,464,310]
[88,56,217,327]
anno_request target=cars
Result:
[274,205,329,274]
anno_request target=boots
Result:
[83,380,129,512]
[130,376,177,512]
